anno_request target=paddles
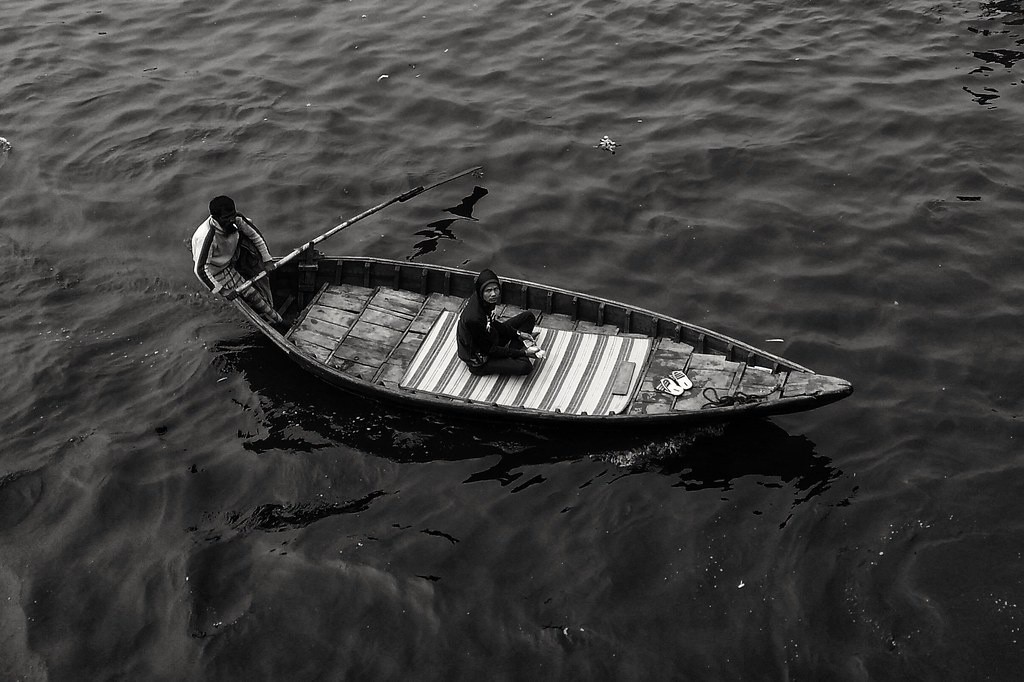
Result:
[235,165,483,294]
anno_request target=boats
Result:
[226,253,856,423]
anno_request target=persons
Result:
[191,196,292,331]
[457,269,540,377]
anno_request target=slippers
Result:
[652,369,693,396]
